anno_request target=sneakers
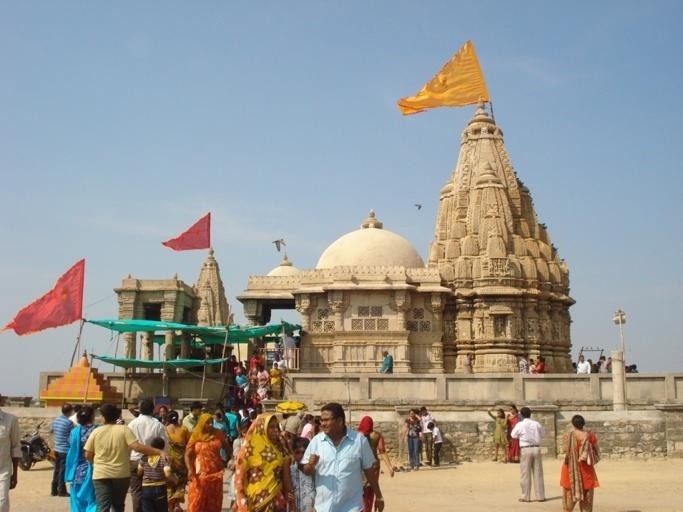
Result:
[538,498,546,503]
[50,490,70,497]
[518,498,531,503]
[406,460,441,473]
[491,457,520,464]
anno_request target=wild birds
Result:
[273,238,286,252]
[414,204,422,210]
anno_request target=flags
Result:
[2,259,85,334]
[161,212,210,251]
[396,40,489,115]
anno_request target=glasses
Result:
[319,415,336,425]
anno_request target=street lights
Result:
[612,309,626,353]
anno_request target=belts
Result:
[520,444,540,449]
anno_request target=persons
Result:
[507,404,521,461]
[487,407,508,463]
[561,414,600,512]
[510,407,547,502]
[572,354,639,374]
[528,358,536,374]
[0,395,22,512]
[532,356,546,373]
[519,354,529,374]
[401,407,444,471]
[463,352,476,375]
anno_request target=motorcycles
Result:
[17,420,54,469]
